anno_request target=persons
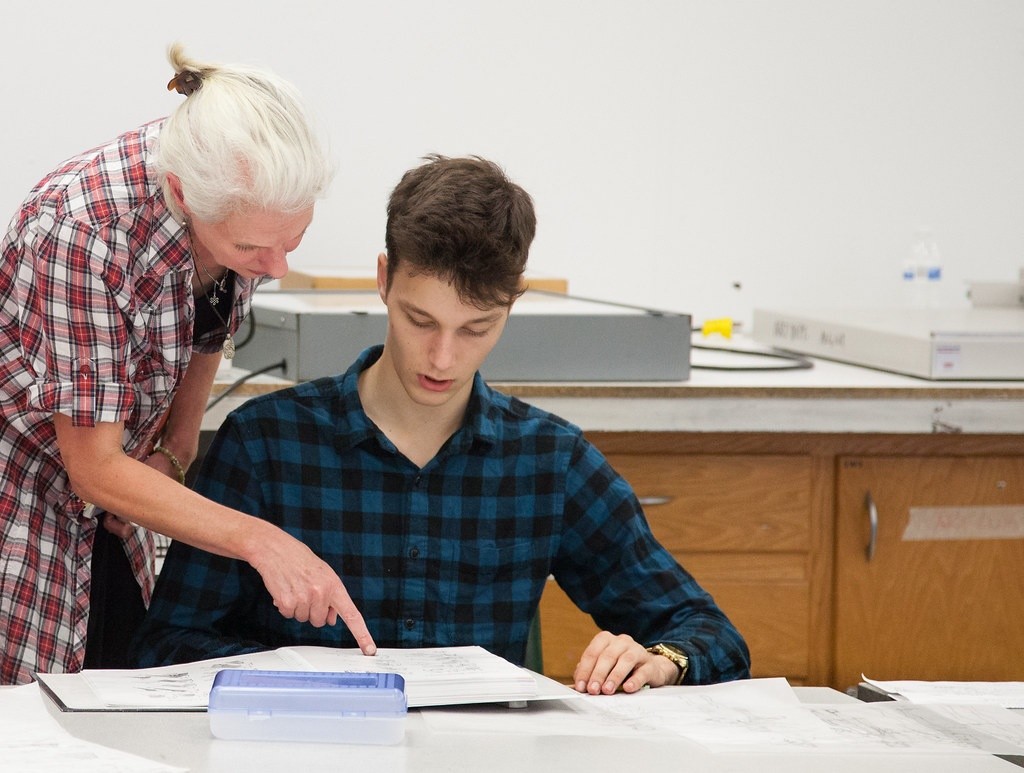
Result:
[0,45,376,685]
[119,158,752,695]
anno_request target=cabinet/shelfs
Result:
[827,431,1024,686]
[532,434,828,691]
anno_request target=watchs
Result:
[647,643,689,685]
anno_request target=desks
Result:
[3,682,1024,773]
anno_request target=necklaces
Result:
[183,220,236,360]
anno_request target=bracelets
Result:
[155,447,186,485]
